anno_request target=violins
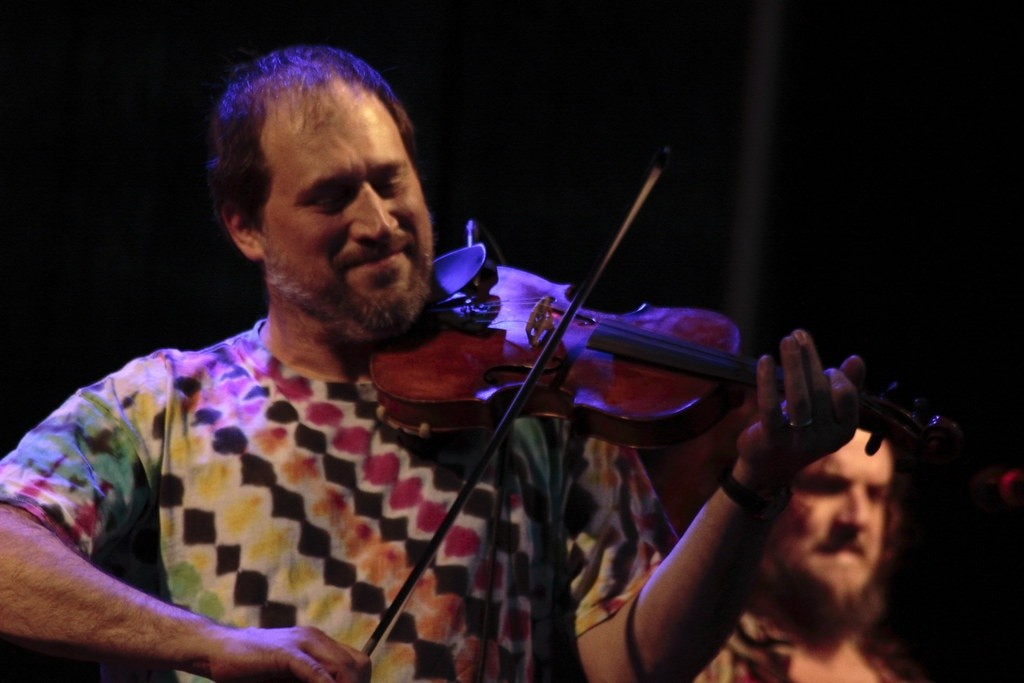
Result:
[368,262,964,466]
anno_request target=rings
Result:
[792,417,814,431]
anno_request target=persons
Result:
[679,402,920,683]
[0,44,862,683]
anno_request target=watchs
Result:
[721,463,791,522]
